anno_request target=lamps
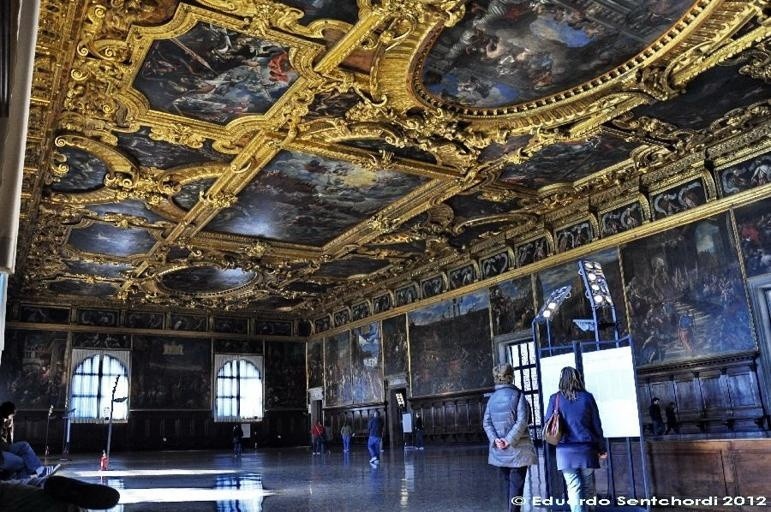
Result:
[533,260,621,342]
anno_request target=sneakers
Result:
[313,452,320,456]
[370,457,379,464]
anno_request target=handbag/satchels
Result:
[542,412,563,445]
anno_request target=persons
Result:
[367,464,384,508]
[625,272,741,364]
[310,419,327,457]
[339,420,353,454]
[231,424,243,459]
[343,454,351,478]
[414,412,425,450]
[1,399,61,488]
[365,410,383,464]
[664,400,680,435]
[544,365,609,512]
[97,448,108,471]
[481,362,537,512]
[324,420,337,457]
[647,396,664,441]
[414,452,424,480]
